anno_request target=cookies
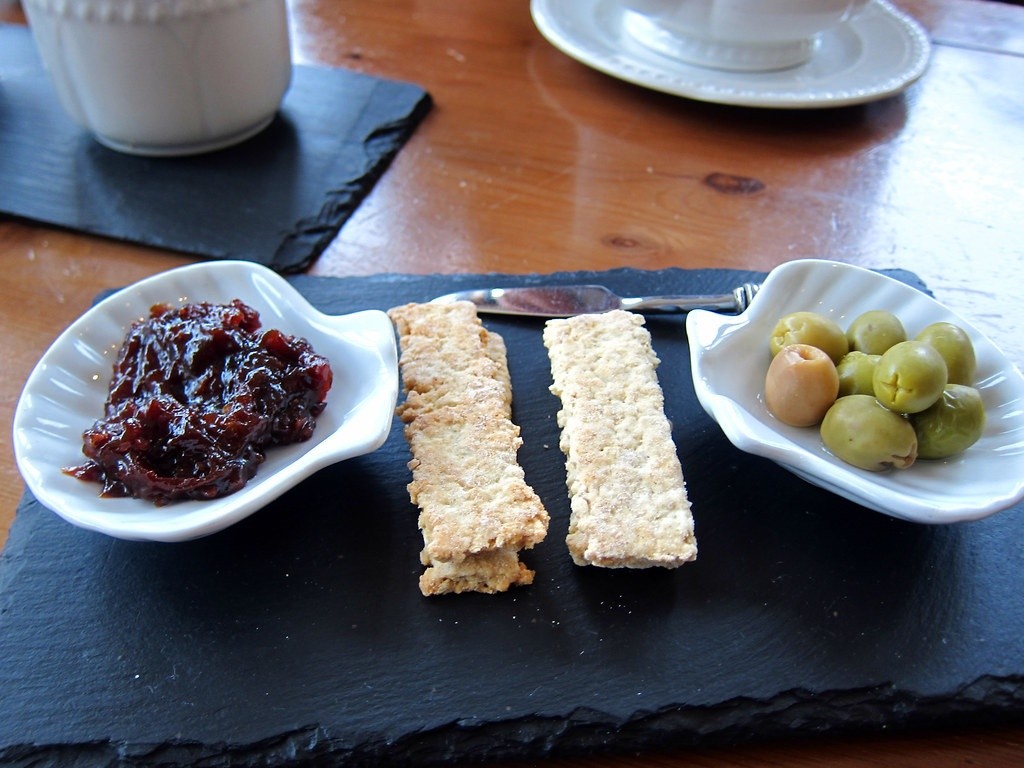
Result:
[387,298,698,595]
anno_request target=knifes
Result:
[412,284,764,318]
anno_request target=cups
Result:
[16,0,294,158]
[625,0,850,72]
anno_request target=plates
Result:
[683,259,1021,523]
[526,0,932,108]
[12,257,406,542]
